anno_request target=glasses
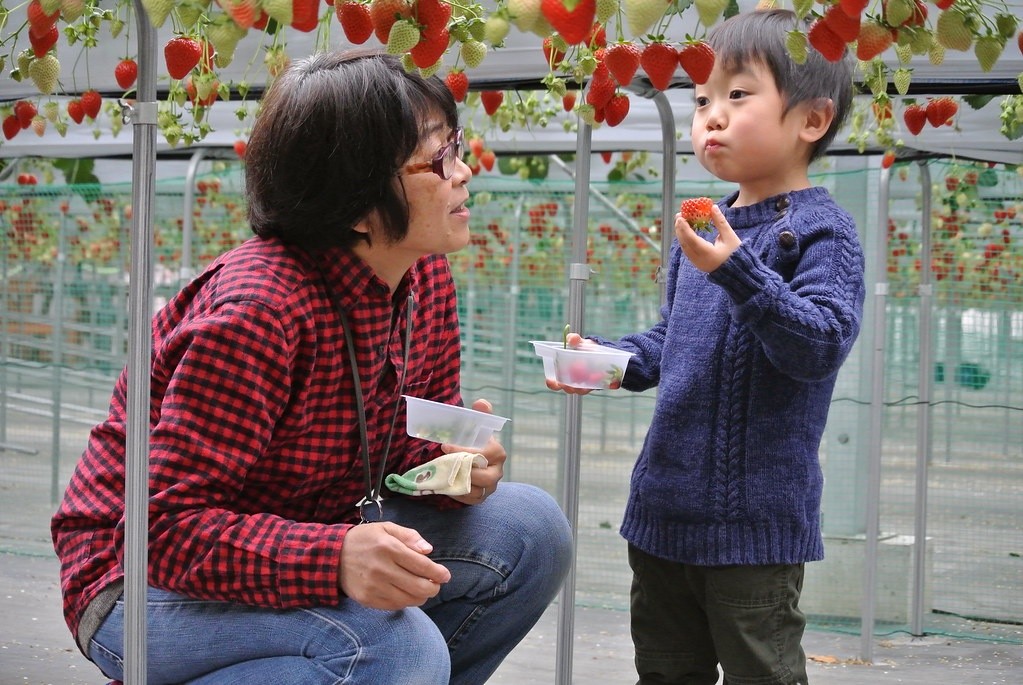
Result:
[389,126,467,182]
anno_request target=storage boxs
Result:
[529,339,637,391]
[401,394,510,449]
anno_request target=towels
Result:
[384,452,488,497]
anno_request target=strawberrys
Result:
[568,357,622,389]
[0,0,1023,302]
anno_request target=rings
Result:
[478,488,485,499]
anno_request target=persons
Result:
[545,9,865,685]
[51,48,576,685]
